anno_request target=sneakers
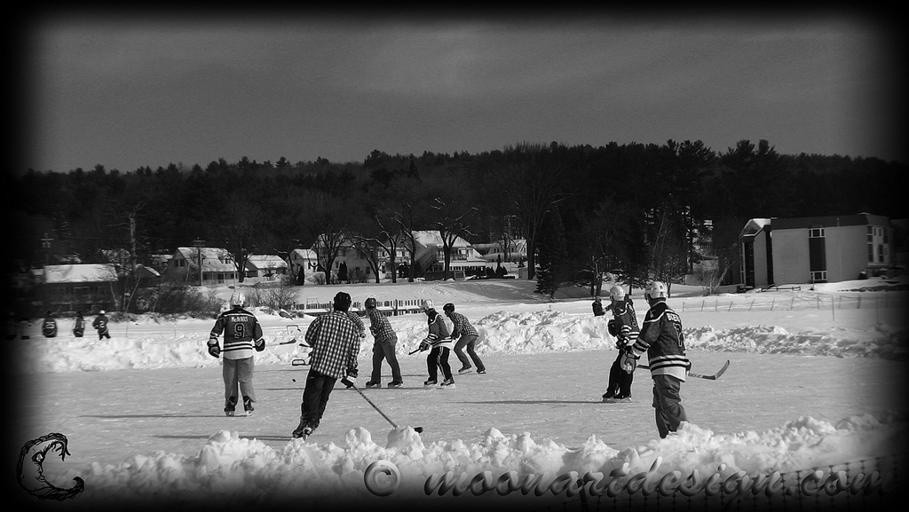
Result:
[458,364,472,375]
[424,380,438,388]
[365,380,382,390]
[476,367,487,375]
[613,391,634,404]
[387,379,404,389]
[440,378,456,389]
[293,421,308,438]
[223,401,236,417]
[302,424,313,442]
[602,390,617,403]
[243,399,255,417]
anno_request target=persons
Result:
[291,292,361,440]
[422,298,455,386]
[71,313,85,337]
[93,310,111,340]
[592,280,692,439]
[207,290,265,417]
[42,310,57,337]
[7,311,33,340]
[365,297,403,388]
[443,303,486,374]
[348,310,366,338]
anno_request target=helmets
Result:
[645,280,666,302]
[333,292,352,312]
[100,309,106,315]
[609,286,626,301]
[443,303,455,312]
[365,298,377,309]
[420,299,433,311]
[230,292,246,307]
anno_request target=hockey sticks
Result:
[219,338,296,352]
[635,359,730,380]
[299,343,314,348]
[352,384,422,433]
[409,349,424,354]
